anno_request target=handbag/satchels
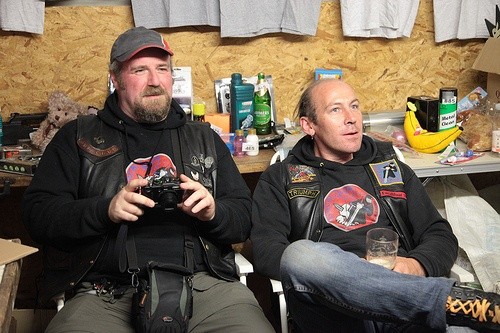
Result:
[130,270,192,333]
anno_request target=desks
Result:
[0,239,23,333]
[404,151,500,188]
[0,149,276,201]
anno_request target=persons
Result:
[19,26,278,333]
[248,75,500,333]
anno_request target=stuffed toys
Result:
[31,89,88,153]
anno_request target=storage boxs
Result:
[204,113,230,133]
[470,36,500,104]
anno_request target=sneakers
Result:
[445,284,500,333]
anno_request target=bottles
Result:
[192,103,205,123]
[229,73,255,132]
[439,87,458,152]
[253,73,272,133]
[233,130,246,156]
[246,128,259,156]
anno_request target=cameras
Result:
[140,175,185,210]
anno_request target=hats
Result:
[110,26,174,65]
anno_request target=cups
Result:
[365,228,400,271]
